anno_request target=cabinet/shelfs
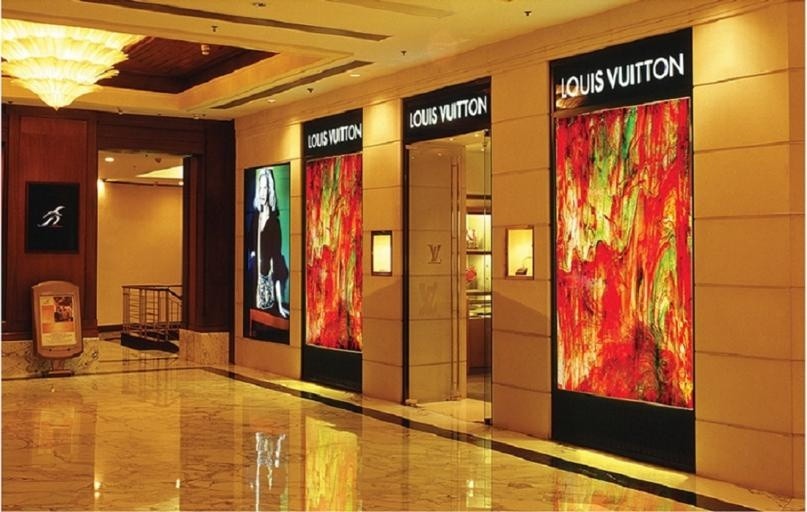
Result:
[465,206,492,375]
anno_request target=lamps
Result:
[1,17,148,111]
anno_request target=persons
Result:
[246,167,291,320]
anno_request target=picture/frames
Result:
[23,180,81,257]
